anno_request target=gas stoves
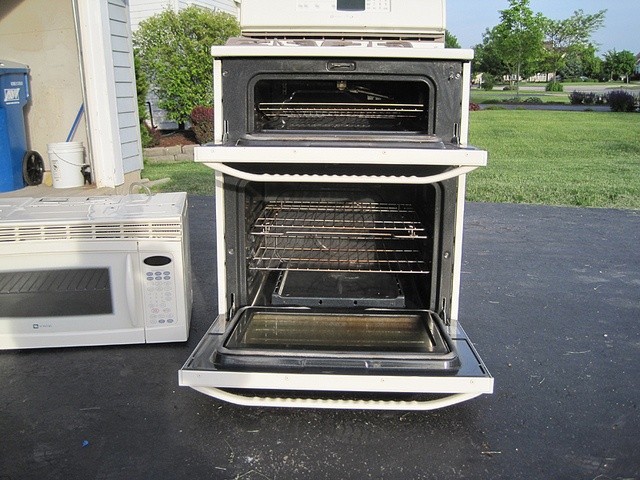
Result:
[210,0,474,62]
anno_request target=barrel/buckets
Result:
[46,140,86,190]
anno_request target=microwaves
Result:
[0,192,195,351]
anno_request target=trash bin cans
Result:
[0,59,42,191]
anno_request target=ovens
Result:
[178,38,494,413]
